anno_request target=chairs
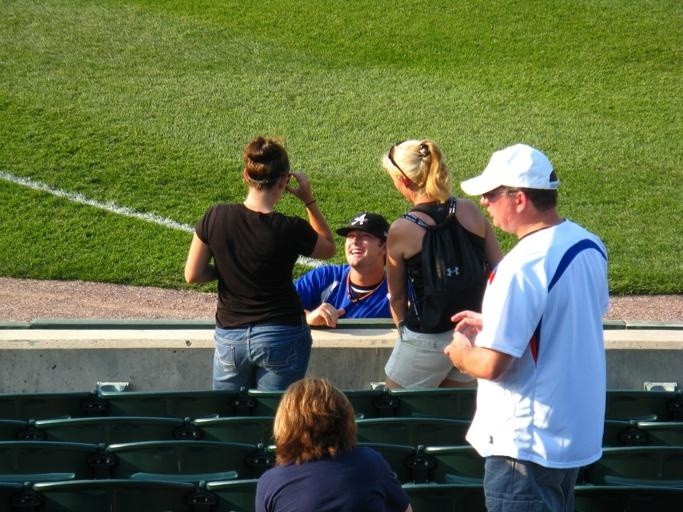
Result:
[0,389,683,512]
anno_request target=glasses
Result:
[389,141,415,184]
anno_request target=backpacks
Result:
[402,197,490,332]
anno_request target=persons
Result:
[377,139,506,396]
[180,134,340,396]
[254,376,413,509]
[442,142,609,511]
[282,209,392,331]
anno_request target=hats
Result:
[461,143,559,196]
[337,212,391,240]
